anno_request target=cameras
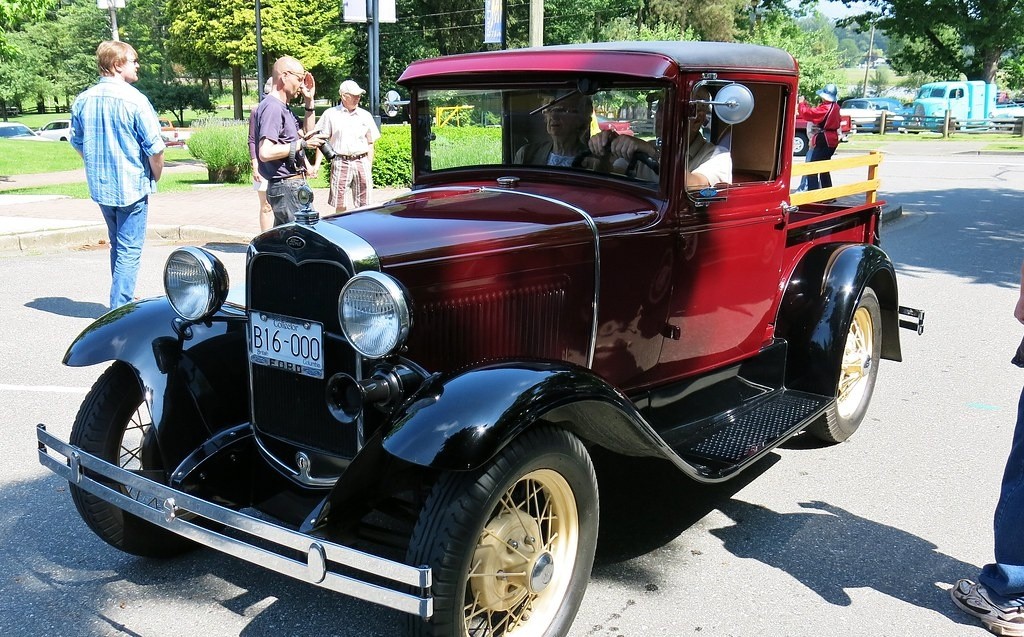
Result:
[306,130,338,163]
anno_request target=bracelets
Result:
[304,106,316,111]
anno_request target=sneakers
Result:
[950,578,1024,636]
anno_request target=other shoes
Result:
[822,198,837,204]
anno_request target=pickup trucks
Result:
[793,112,852,158]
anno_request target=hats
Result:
[816,84,839,102]
[339,80,366,97]
[646,85,713,116]
[263,77,273,94]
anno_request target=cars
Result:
[839,99,903,134]
[861,97,916,133]
[0,122,55,141]
[36,41,929,637]
[593,113,636,139]
[33,120,73,143]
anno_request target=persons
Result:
[69,41,166,311]
[792,99,825,193]
[797,83,843,204]
[587,85,732,190]
[512,91,600,170]
[247,77,276,232]
[949,256,1023,637]
[315,79,381,213]
[255,57,329,228]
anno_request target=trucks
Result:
[909,80,1024,134]
[157,116,198,148]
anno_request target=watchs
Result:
[302,138,306,150]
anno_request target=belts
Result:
[269,171,306,181]
[336,152,369,161]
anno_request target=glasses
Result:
[285,70,306,84]
[125,57,138,67]
[541,104,580,118]
[649,102,664,112]
